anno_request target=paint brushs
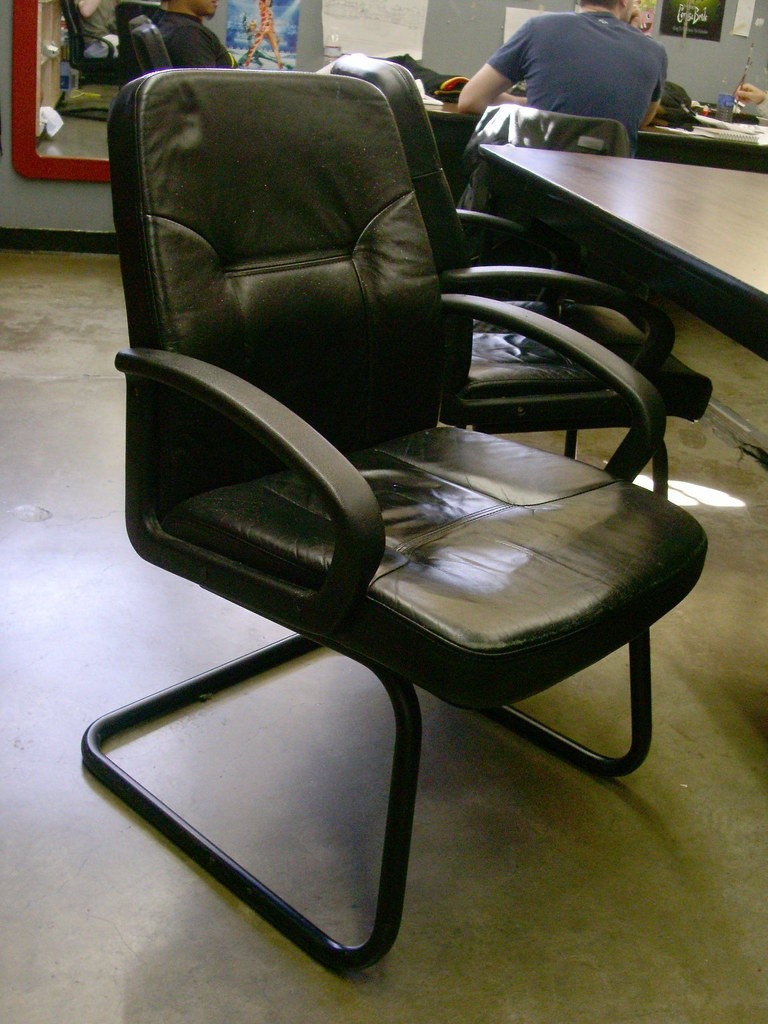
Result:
[732,41,755,116]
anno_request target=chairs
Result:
[327,54,713,498]
[74,70,709,978]
[131,24,173,77]
[128,13,152,30]
[457,103,633,310]
[55,0,121,121]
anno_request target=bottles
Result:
[715,78,735,123]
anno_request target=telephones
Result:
[434,77,470,98]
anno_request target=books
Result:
[692,125,759,144]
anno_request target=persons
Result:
[457,0,668,237]
[736,83,768,118]
[74,0,122,61]
[158,0,240,70]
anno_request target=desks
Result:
[420,92,768,153]
[476,138,768,367]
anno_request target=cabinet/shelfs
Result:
[36,2,61,136]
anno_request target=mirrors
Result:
[11,0,110,184]
